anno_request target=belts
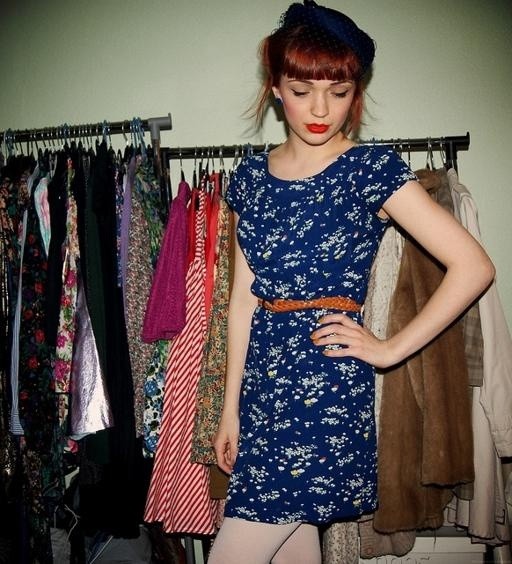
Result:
[257,295,363,314]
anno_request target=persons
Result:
[208,0,496,564]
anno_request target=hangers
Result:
[0,114,150,159]
[149,132,463,189]
